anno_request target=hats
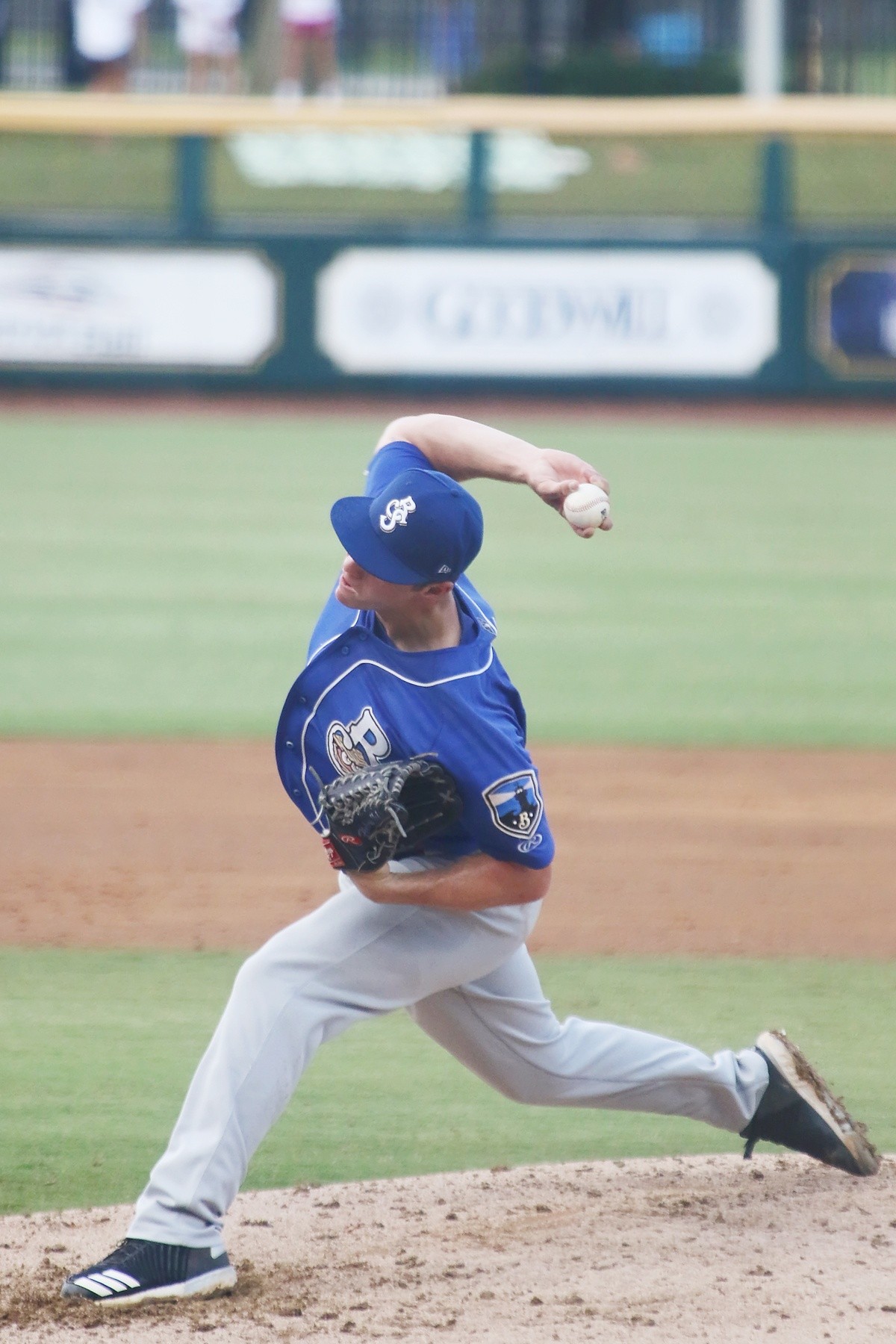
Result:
[330,470,483,585]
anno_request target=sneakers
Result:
[58,1238,237,1307]
[739,1029,880,1176]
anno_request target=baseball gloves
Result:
[320,748,468,873]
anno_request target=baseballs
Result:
[563,482,610,528]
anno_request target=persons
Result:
[55,412,885,1313]
[61,0,347,96]
